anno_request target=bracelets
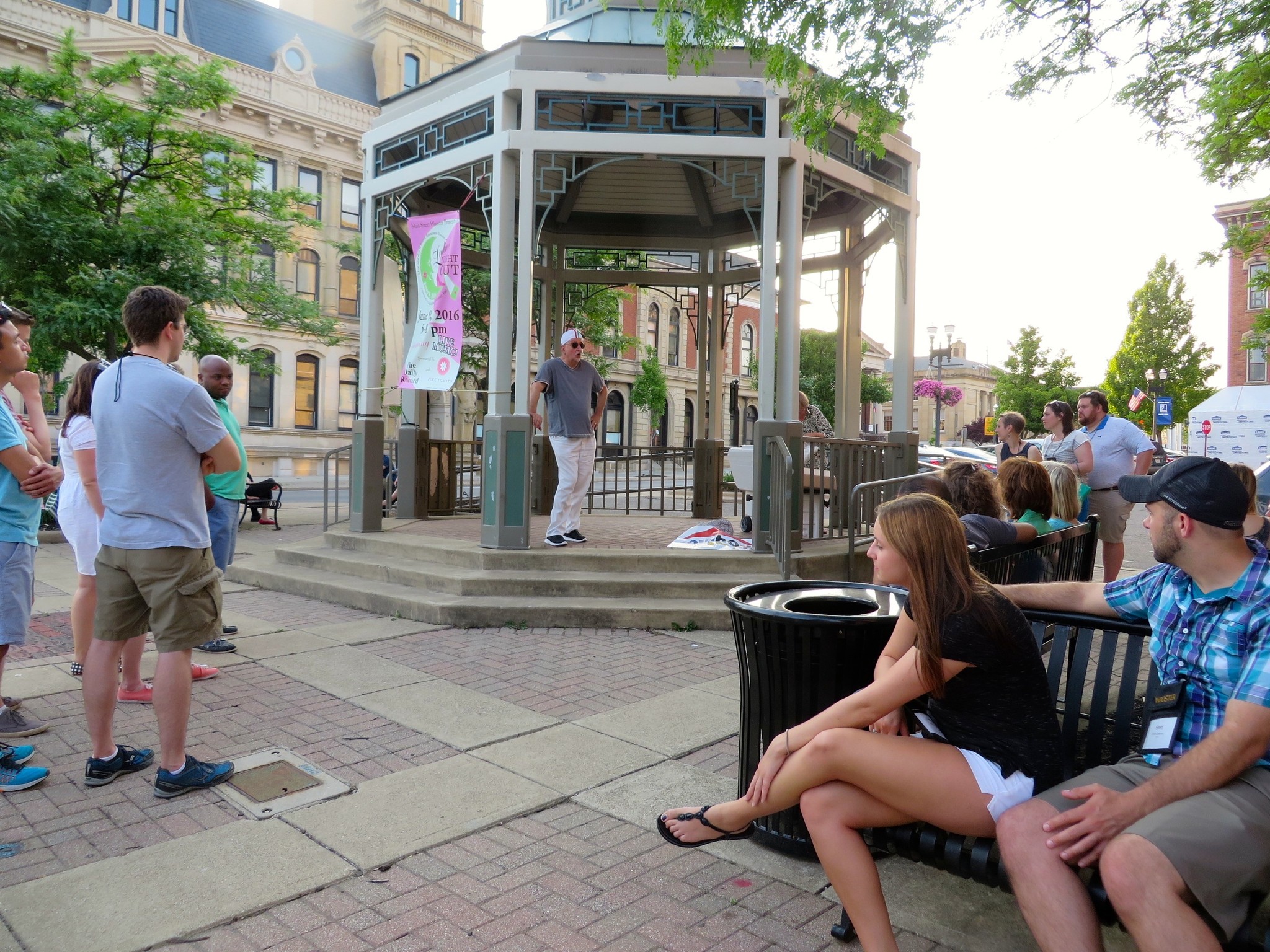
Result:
[785,729,792,755]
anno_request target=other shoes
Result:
[119,663,122,673]
[70,661,84,675]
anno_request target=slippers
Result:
[656,805,755,848]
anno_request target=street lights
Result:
[1145,367,1168,443]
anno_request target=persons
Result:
[993,410,1043,470]
[1075,390,1157,582]
[1228,462,1270,552]
[657,492,1061,948]
[1039,460,1081,531]
[115,623,219,705]
[939,461,1005,519]
[82,284,236,798]
[191,355,242,653]
[57,359,127,676]
[249,453,398,524]
[0,302,54,736]
[798,391,835,471]
[1040,399,1094,522]
[898,474,1038,548]
[984,454,1268,951]
[0,307,64,790]
[995,456,1053,532]
[528,328,609,545]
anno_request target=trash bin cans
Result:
[723,580,909,864]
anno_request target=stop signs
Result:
[1202,421,1212,434]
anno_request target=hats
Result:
[1118,456,1249,530]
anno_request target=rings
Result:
[595,423,597,425]
[873,728,879,734]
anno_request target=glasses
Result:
[161,319,191,336]
[93,359,112,388]
[1085,391,1103,400]
[0,301,13,312]
[564,342,585,349]
[1044,400,1062,413]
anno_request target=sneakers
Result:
[220,625,239,635]
[0,745,35,764]
[192,637,238,654]
[153,754,235,797]
[563,530,587,542]
[117,683,153,703]
[84,744,155,785]
[0,696,23,709]
[544,535,568,546]
[191,662,220,681]
[0,707,50,738]
[0,750,51,791]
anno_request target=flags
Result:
[396,208,461,392]
[1128,386,1146,412]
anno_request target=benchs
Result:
[828,608,1269,952]
[239,482,281,530]
[970,511,1101,689]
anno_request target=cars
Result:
[861,446,1046,506]
[1133,439,1188,473]
